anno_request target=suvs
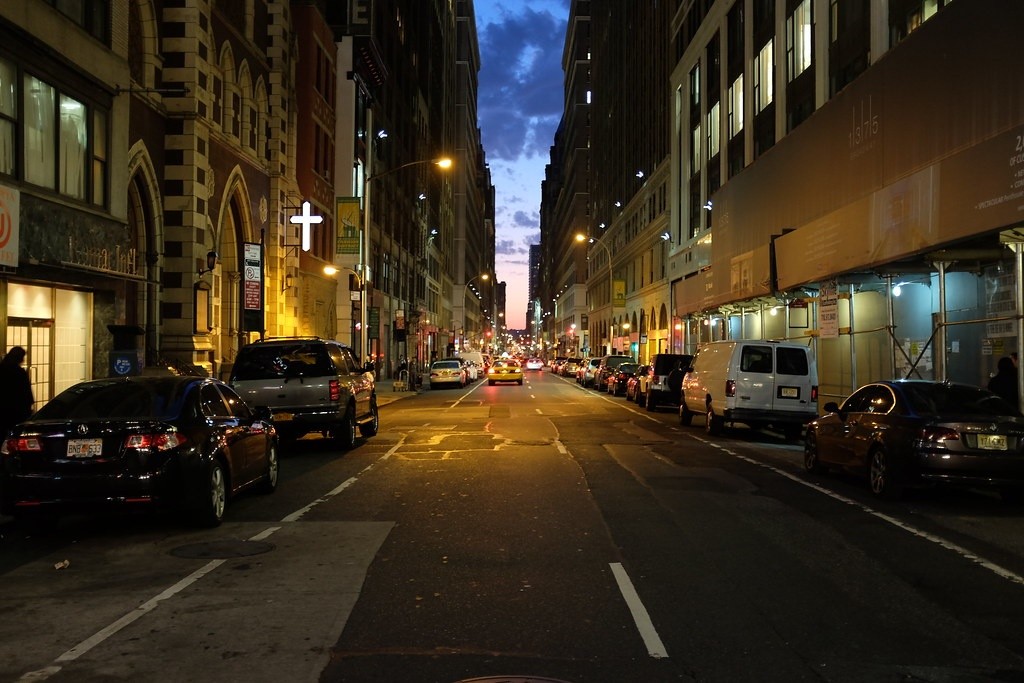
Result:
[638,353,695,412]
[229,335,380,453]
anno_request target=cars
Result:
[429,360,467,391]
[440,352,530,385]
[0,375,280,540]
[804,379,1024,504]
[550,354,651,404]
[526,358,544,371]
[487,356,524,386]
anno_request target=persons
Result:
[396,354,424,392]
[0,346,34,445]
[985,357,1018,403]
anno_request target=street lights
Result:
[575,234,613,355]
[322,155,454,371]
[462,273,489,350]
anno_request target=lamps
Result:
[199,249,218,278]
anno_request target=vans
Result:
[678,339,819,443]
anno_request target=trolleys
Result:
[392,370,410,392]
